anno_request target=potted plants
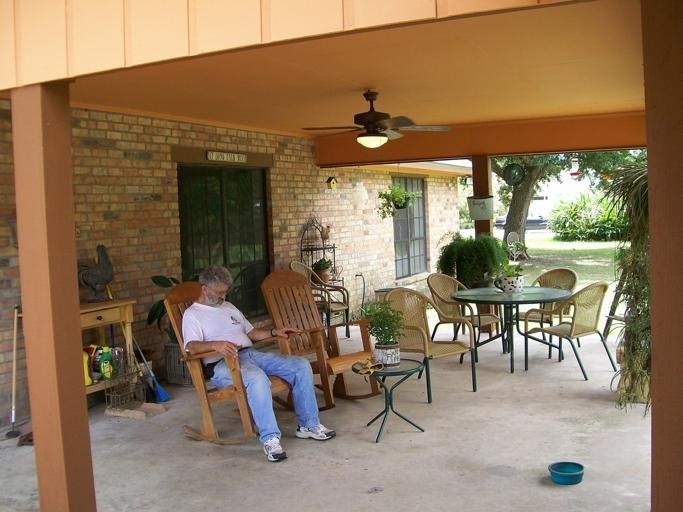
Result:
[375,177,423,223]
[491,261,526,295]
[435,228,510,291]
[599,149,651,421]
[144,270,202,389]
[354,291,407,367]
[309,258,335,285]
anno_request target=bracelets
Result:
[270,327,277,337]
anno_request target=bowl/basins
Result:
[548,462,584,484]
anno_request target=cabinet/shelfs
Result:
[298,216,352,343]
[74,296,138,407]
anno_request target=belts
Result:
[207,346,252,369]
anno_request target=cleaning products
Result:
[82,350,92,386]
[99,347,118,382]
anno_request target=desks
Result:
[447,282,574,376]
[351,356,430,444]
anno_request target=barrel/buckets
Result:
[375,340,401,369]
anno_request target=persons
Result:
[181,263,338,462]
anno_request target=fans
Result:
[295,87,451,141]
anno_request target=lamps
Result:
[464,194,494,221]
[354,128,389,150]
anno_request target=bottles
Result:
[98,346,113,381]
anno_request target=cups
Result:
[110,347,123,375]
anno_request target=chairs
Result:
[162,278,298,448]
[520,280,618,382]
[254,268,383,411]
[288,259,351,347]
[506,266,579,360]
[382,286,479,404]
[505,231,529,263]
[424,271,506,366]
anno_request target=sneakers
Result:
[262,436,288,463]
[295,424,336,442]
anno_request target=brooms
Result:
[94,256,156,402]
[132,335,170,403]
